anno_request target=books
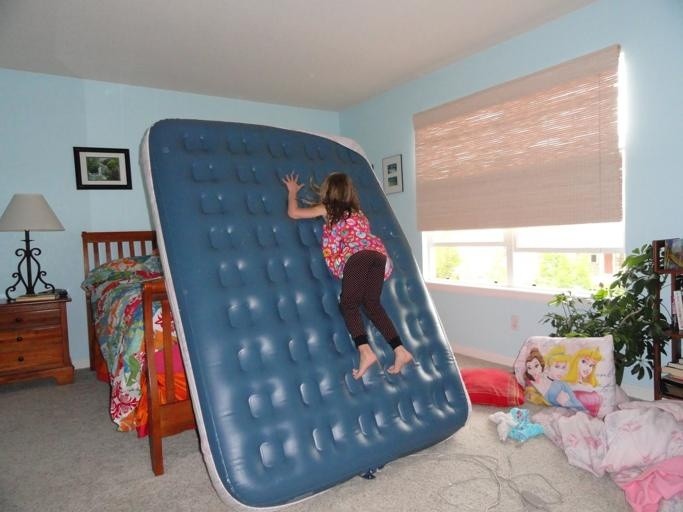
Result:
[659,354,682,386]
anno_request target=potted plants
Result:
[541,242,673,390]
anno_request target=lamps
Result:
[0,193,65,303]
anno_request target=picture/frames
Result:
[73,146,133,191]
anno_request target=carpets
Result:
[2,348,678,511]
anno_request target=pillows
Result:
[513,336,616,391]
[459,369,524,407]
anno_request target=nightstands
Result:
[0,297,76,386]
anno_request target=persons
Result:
[523,345,604,416]
[281,172,413,380]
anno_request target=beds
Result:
[81,228,202,477]
